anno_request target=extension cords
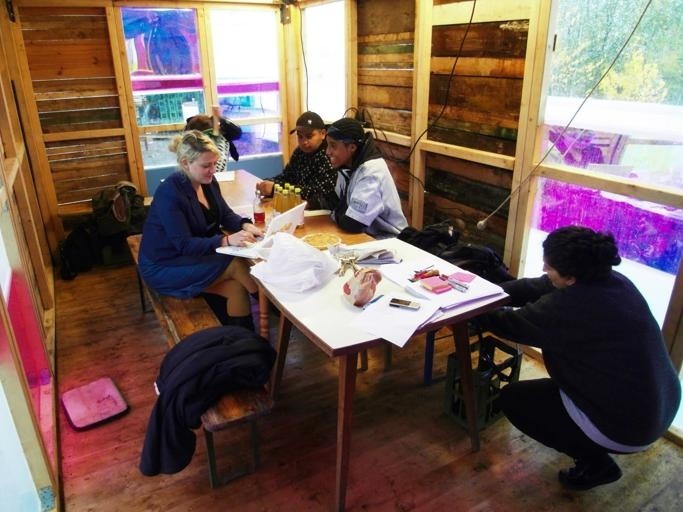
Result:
[355,120,371,128]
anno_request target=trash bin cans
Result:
[447,335,522,432]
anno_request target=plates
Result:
[300,232,342,248]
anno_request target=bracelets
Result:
[226,234,231,246]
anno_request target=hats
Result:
[289,111,324,135]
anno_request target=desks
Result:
[160,169,512,512]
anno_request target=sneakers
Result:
[556,458,624,490]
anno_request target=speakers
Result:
[281,4,291,23]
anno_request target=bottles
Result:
[253,189,266,223]
[273,182,303,229]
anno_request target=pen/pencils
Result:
[439,274,469,292]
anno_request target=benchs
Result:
[126,235,263,487]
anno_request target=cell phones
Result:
[389,298,421,310]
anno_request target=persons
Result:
[183,104,242,161]
[255,112,337,211]
[138,131,281,335]
[468,225,681,490]
[324,118,409,238]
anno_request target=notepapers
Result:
[420,276,451,294]
